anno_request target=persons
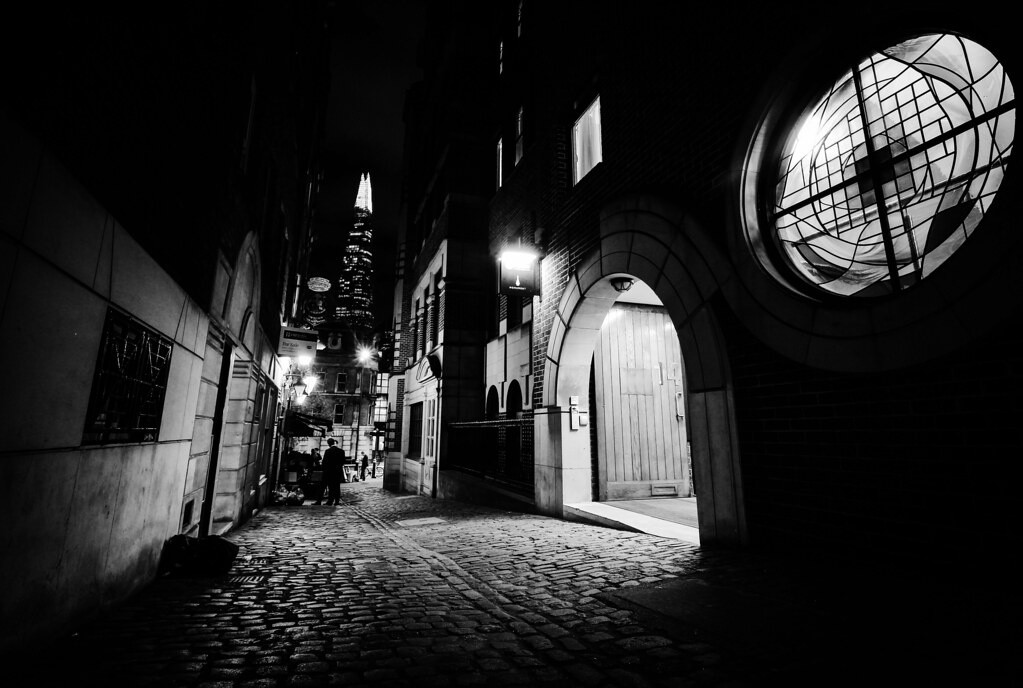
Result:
[360,451,368,481]
[311,438,345,506]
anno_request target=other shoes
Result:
[323,502,333,505]
[311,502,321,505]
[334,503,339,505]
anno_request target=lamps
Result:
[609,277,634,293]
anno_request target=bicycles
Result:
[376,462,384,477]
[358,464,370,478]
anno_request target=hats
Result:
[315,448,320,450]
